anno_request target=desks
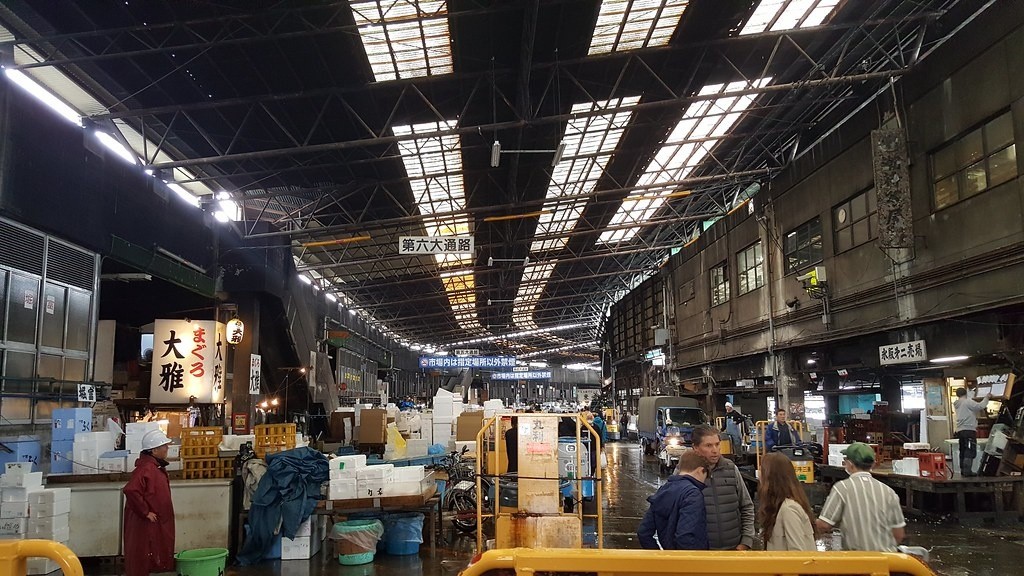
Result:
[943,438,988,474]
[311,491,444,559]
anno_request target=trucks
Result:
[635,395,712,458]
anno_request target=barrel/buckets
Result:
[173,548,229,576]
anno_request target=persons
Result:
[123,430,175,576]
[690,425,755,551]
[953,387,992,477]
[814,442,907,554]
[765,409,803,453]
[757,452,816,552]
[637,449,712,551]
[719,401,745,465]
[504,403,627,513]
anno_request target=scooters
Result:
[655,425,689,474]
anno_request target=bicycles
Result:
[422,443,493,534]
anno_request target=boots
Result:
[960,457,977,477]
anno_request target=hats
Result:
[840,442,874,467]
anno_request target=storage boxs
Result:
[892,458,921,477]
[749,434,763,441]
[900,448,914,457]
[867,432,884,444]
[871,445,880,458]
[884,446,893,450]
[751,442,761,446]
[827,455,847,466]
[917,451,946,478]
[608,432,620,439]
[882,452,891,458]
[607,424,620,432]
[903,442,930,449]
[828,443,850,455]
[516,415,559,479]
[895,446,902,454]
[751,428,763,434]
[517,477,559,514]
[0,386,512,576]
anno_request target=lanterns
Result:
[226,317,244,345]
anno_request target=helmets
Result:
[140,431,172,450]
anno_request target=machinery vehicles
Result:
[735,420,825,507]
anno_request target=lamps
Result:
[487,221,494,267]
[552,49,568,167]
[490,55,500,168]
[488,273,491,305]
[513,299,519,305]
[807,356,815,364]
[523,219,530,266]
